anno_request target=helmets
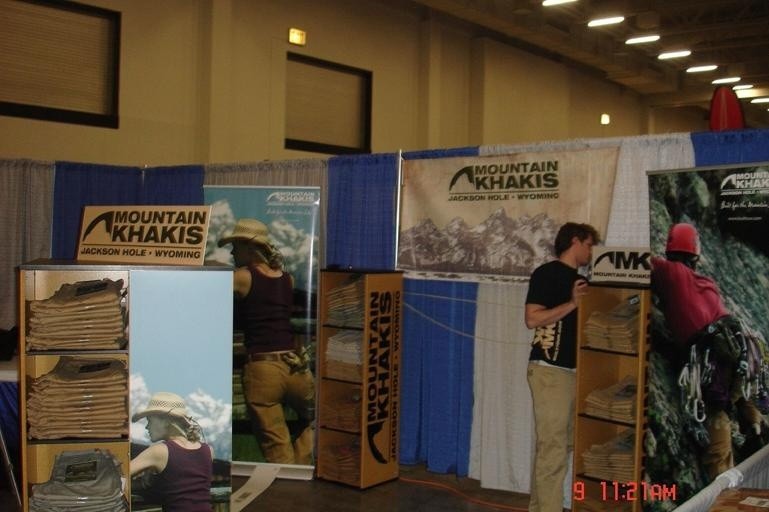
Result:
[667,223,701,256]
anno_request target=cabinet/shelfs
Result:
[315,268,405,490]
[573,283,652,511]
[20,258,236,512]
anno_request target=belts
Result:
[250,350,299,361]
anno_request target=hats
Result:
[132,392,196,425]
[218,217,276,254]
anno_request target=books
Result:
[581,293,638,487]
[321,276,365,484]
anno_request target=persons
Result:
[218,219,316,468]
[130,393,215,511]
[523,222,601,512]
[652,223,768,480]
